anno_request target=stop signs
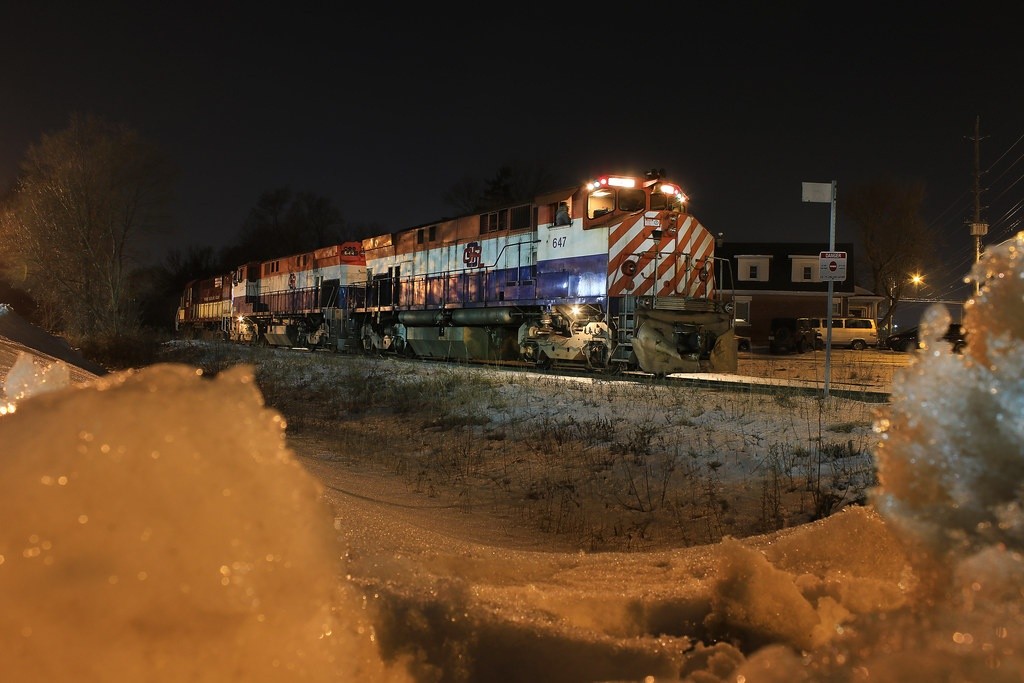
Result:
[829,261,838,272]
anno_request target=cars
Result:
[884,323,965,354]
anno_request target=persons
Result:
[555,201,571,226]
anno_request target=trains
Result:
[173,169,738,375]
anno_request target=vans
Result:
[808,317,878,350]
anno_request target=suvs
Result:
[769,316,823,355]
